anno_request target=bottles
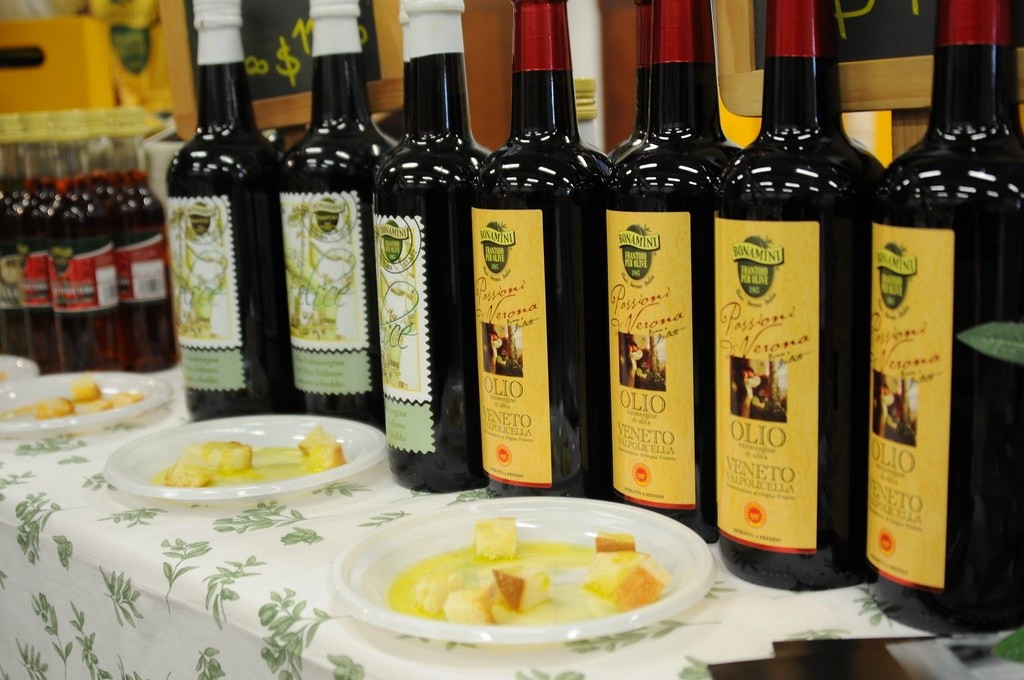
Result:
[0,106,180,375]
[158,0,1024,635]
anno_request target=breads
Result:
[35,378,144,419]
[162,426,346,487]
[414,513,668,627]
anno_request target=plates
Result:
[0,354,40,385]
[101,413,389,510]
[0,372,173,436]
[329,494,718,649]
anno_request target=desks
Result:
[0,366,942,680]
